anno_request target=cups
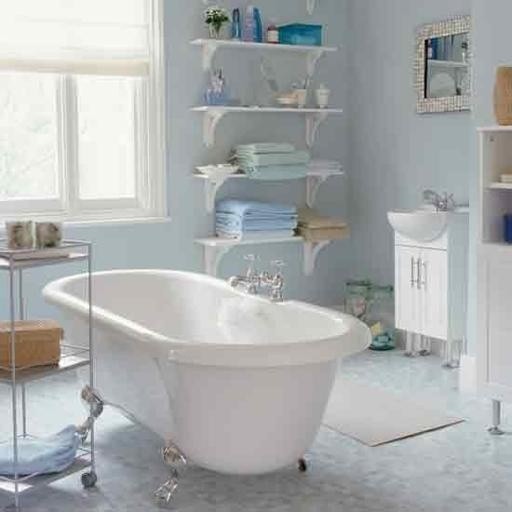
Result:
[5,219,33,248]
[274,86,336,108]
[33,221,63,248]
[499,174,512,184]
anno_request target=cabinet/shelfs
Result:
[474,124,512,436]
[0,239,96,512]
[393,241,470,366]
[187,36,349,278]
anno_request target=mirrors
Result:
[410,13,473,115]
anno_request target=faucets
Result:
[421,189,455,211]
[229,254,287,303]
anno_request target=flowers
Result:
[204,6,230,32]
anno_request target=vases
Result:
[208,23,220,38]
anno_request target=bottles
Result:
[230,5,280,45]
[427,40,433,57]
[492,66,512,125]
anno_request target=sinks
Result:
[386,204,469,243]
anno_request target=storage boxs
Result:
[0,318,66,372]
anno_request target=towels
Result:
[0,423,80,475]
[214,139,348,245]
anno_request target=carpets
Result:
[318,368,468,448]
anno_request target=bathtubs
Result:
[41,267,373,509]
